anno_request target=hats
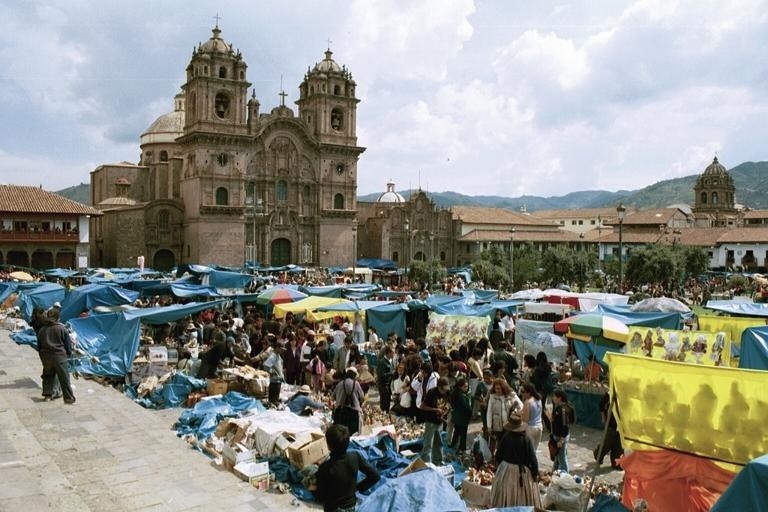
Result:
[53,301,64,309]
[418,348,432,362]
[346,365,360,379]
[298,385,311,394]
[503,413,528,433]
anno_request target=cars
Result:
[700,268,768,284]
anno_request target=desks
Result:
[565,389,605,431]
[229,414,324,459]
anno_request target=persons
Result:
[2,224,77,234]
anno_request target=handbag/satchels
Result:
[547,435,561,461]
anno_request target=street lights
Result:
[614,201,627,292]
[507,224,516,291]
[351,226,356,283]
[596,212,603,270]
[672,227,682,248]
[402,217,412,286]
[242,196,265,277]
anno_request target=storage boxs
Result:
[233,461,269,492]
[222,442,257,472]
[132,346,179,385]
[288,432,329,469]
[208,377,228,396]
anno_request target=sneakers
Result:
[592,449,625,472]
